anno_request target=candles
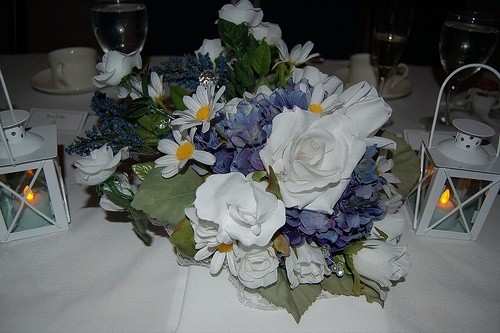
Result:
[13,190,53,228]
[426,200,460,227]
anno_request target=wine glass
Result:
[372,23,406,95]
[418,4,500,129]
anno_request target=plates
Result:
[344,75,411,100]
[31,68,95,95]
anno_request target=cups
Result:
[348,54,409,90]
[89,0,147,56]
[48,48,97,90]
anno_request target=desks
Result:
[0,52,500,333]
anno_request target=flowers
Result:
[65,0,422,322]
